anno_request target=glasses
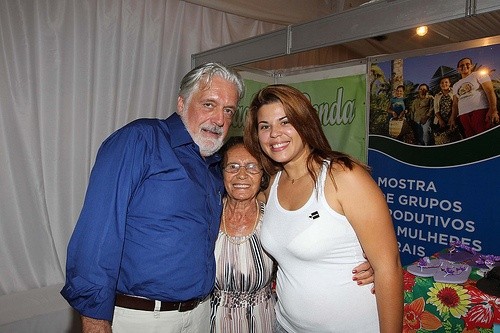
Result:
[222,163,264,174]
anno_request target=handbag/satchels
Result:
[389,118,407,138]
[434,128,462,145]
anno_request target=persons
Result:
[207,136,376,333]
[411,83,435,147]
[78,63,246,333]
[433,76,454,134]
[387,85,409,139]
[447,57,499,138]
[243,83,404,333]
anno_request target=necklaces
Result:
[283,172,309,184]
[222,197,260,244]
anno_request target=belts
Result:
[115,292,208,312]
